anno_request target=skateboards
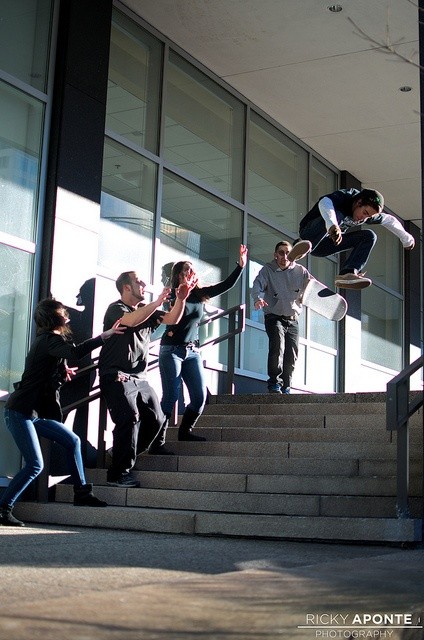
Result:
[297,279,348,322]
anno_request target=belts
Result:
[264,314,298,321]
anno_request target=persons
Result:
[0,295,128,528]
[98,270,198,488]
[287,187,417,290]
[150,243,248,455]
[252,241,315,395]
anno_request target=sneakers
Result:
[287,240,311,263]
[109,474,140,488]
[335,274,371,287]
[150,445,177,455]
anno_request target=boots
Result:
[74,483,106,505]
[0,504,24,527]
[178,409,206,441]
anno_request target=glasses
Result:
[276,251,291,257]
